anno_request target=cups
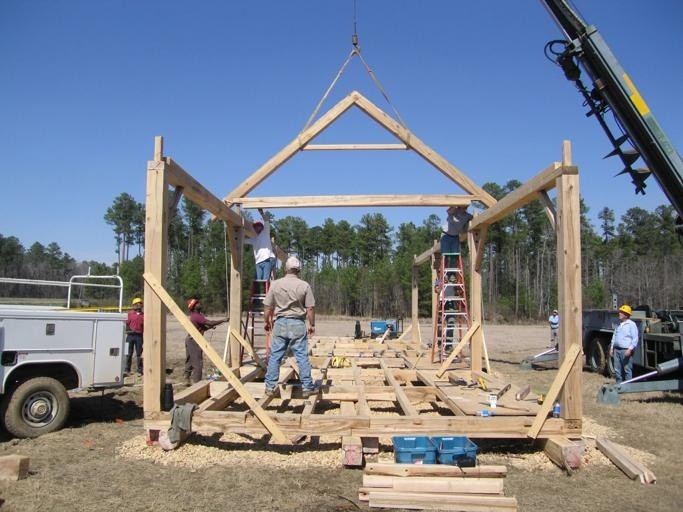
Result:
[486,394,497,408]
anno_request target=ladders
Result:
[431,253,471,366]
[241,279,275,365]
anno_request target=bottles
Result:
[163,383,173,411]
[482,410,488,417]
[552,400,560,417]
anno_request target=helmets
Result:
[131,297,143,305]
[618,305,632,316]
[252,220,260,227]
[186,298,198,310]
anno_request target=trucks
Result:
[0,275,128,438]
[582,303,683,385]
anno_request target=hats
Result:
[285,256,301,272]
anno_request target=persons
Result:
[123,297,144,377]
[262,256,321,397]
[434,270,459,352]
[549,309,559,348]
[184,296,230,383]
[609,305,639,384]
[439,204,475,283]
[237,207,276,305]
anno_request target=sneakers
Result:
[265,384,278,396]
[302,387,318,397]
[449,274,457,282]
[444,275,448,283]
